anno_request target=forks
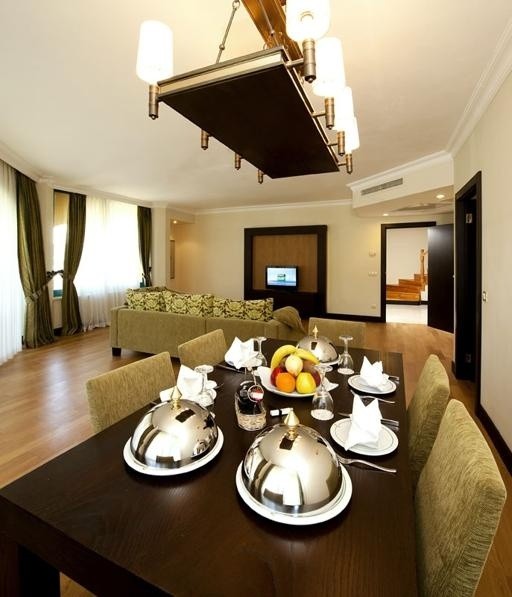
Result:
[347,374,397,395]
[348,386,395,404]
[332,451,398,473]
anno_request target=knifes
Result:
[214,359,246,375]
[335,412,401,425]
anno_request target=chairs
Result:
[84,350,177,435]
[307,316,367,350]
[176,328,230,376]
[406,352,450,487]
[416,396,507,596]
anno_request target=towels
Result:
[342,387,386,451]
[358,351,391,394]
[159,362,218,406]
[224,335,262,370]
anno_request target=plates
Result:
[255,365,339,397]
[315,352,340,367]
[234,460,355,527]
[328,418,398,457]
[122,425,224,478]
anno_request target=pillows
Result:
[213,295,275,323]
[126,291,169,312]
[163,289,214,318]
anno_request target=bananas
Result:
[298,349,319,364]
[270,344,297,368]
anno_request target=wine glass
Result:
[253,335,268,366]
[194,364,216,410]
[311,364,334,420]
[336,334,356,375]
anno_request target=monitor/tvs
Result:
[265,265,299,291]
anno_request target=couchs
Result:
[109,287,306,358]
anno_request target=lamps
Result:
[135,0,361,186]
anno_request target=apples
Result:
[271,367,286,386]
[302,368,320,387]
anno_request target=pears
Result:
[296,372,316,393]
